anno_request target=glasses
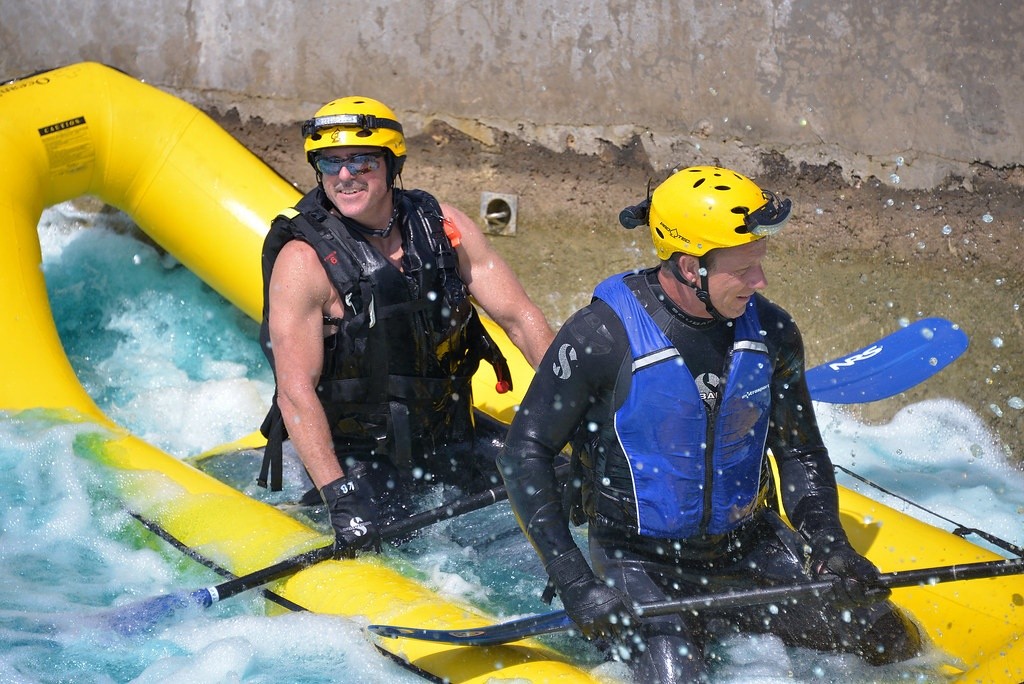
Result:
[313,151,389,176]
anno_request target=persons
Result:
[259,96,556,560]
[496,167,921,684]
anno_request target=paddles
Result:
[367,557,1024,647]
[205,315,971,605]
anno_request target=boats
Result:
[0,61,1024,684]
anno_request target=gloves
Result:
[797,527,892,616]
[539,547,646,663]
[320,474,384,561]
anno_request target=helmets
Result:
[649,165,793,262]
[301,96,408,163]
